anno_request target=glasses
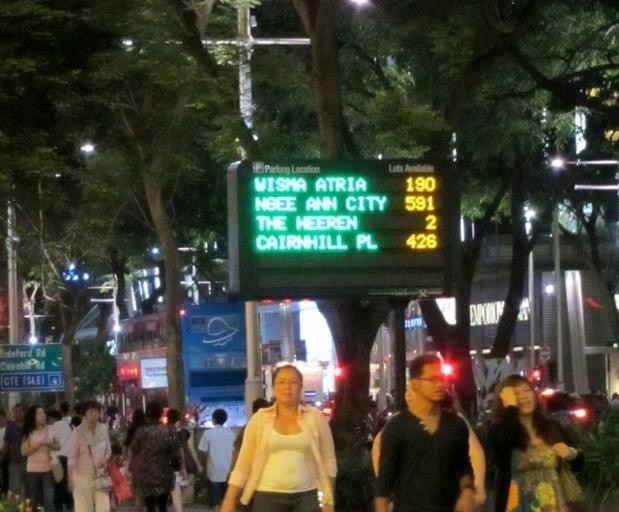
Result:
[418,376,443,382]
[277,380,302,388]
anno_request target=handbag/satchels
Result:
[51,454,65,482]
[94,475,114,491]
[559,466,583,503]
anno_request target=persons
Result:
[0,398,269,511]
[220,350,585,511]
[610,392,619,411]
